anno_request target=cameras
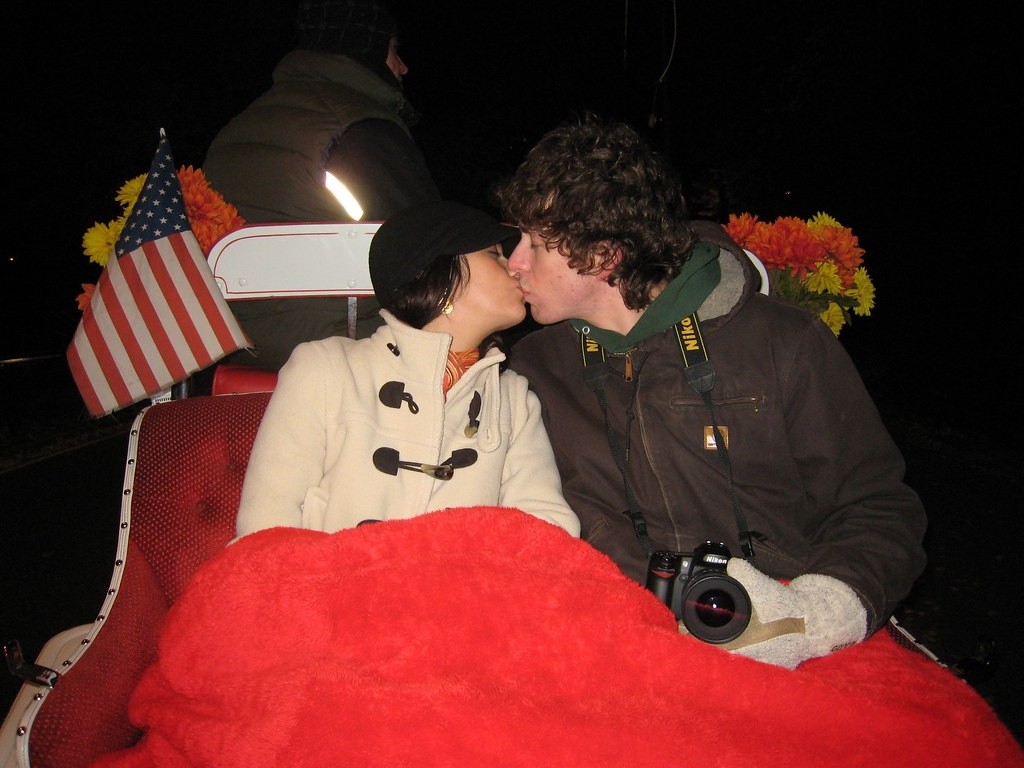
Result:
[647,541,753,644]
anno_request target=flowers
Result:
[74,161,244,314]
[720,210,876,339]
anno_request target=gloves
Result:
[679,557,868,670]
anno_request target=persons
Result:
[498,109,929,672]
[201,1,449,371]
[224,197,582,545]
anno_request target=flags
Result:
[66,138,257,419]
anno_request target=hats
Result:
[295,0,398,62]
[368,200,522,307]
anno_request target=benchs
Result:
[0,390,274,768]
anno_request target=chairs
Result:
[205,220,397,396]
